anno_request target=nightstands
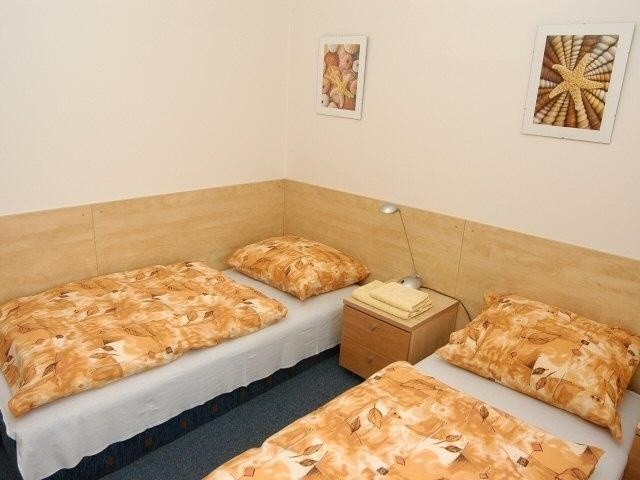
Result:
[338,301,459,383]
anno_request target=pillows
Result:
[227,234,370,301]
[438,293,640,446]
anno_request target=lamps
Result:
[378,203,425,290]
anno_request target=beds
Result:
[199,344,640,480]
[0,268,365,479]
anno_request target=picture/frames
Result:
[315,32,370,122]
[518,18,636,148]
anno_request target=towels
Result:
[352,279,435,322]
